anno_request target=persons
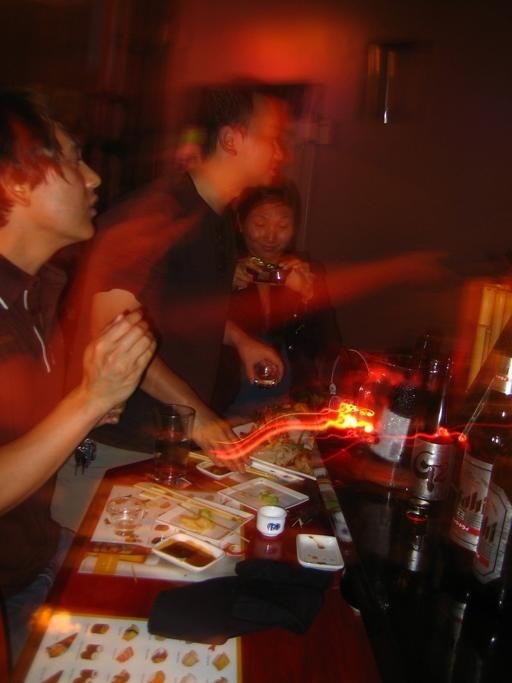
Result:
[0,74,452,668]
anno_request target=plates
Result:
[153,455,346,571]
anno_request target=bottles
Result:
[372,334,434,465]
[443,318,512,570]
[404,358,451,523]
[390,533,510,679]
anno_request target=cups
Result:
[254,363,277,389]
[146,404,195,477]
[106,494,144,537]
